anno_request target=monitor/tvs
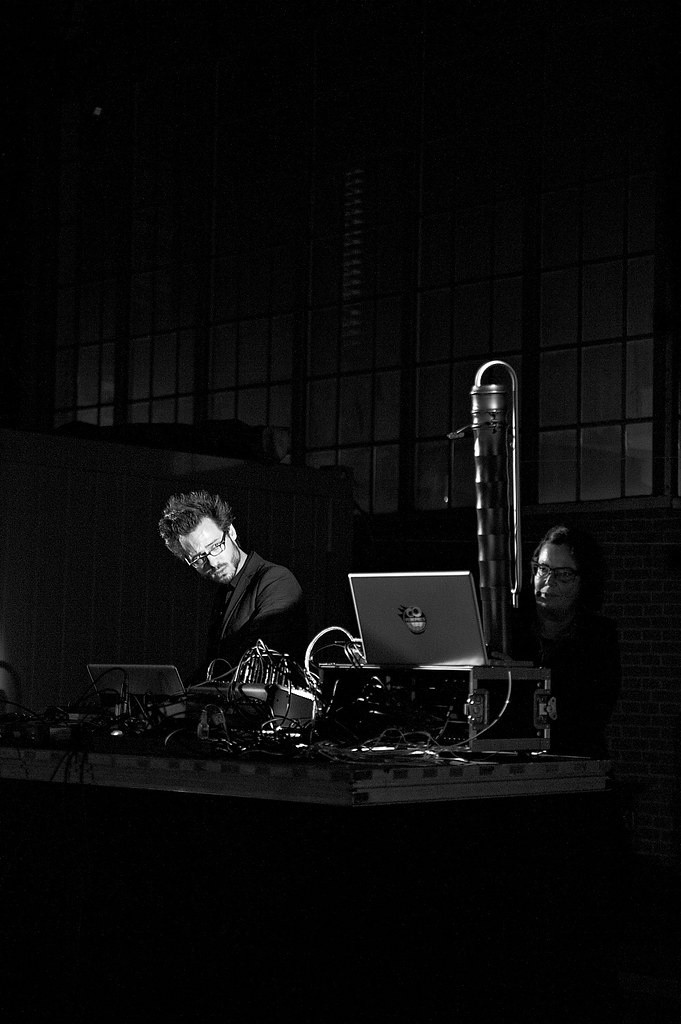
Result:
[87,663,187,719]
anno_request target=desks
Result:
[2,777,647,1023]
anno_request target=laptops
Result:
[348,571,534,667]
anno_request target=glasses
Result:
[184,531,228,569]
[531,562,581,584]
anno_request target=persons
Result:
[158,489,308,682]
[503,523,621,747]
